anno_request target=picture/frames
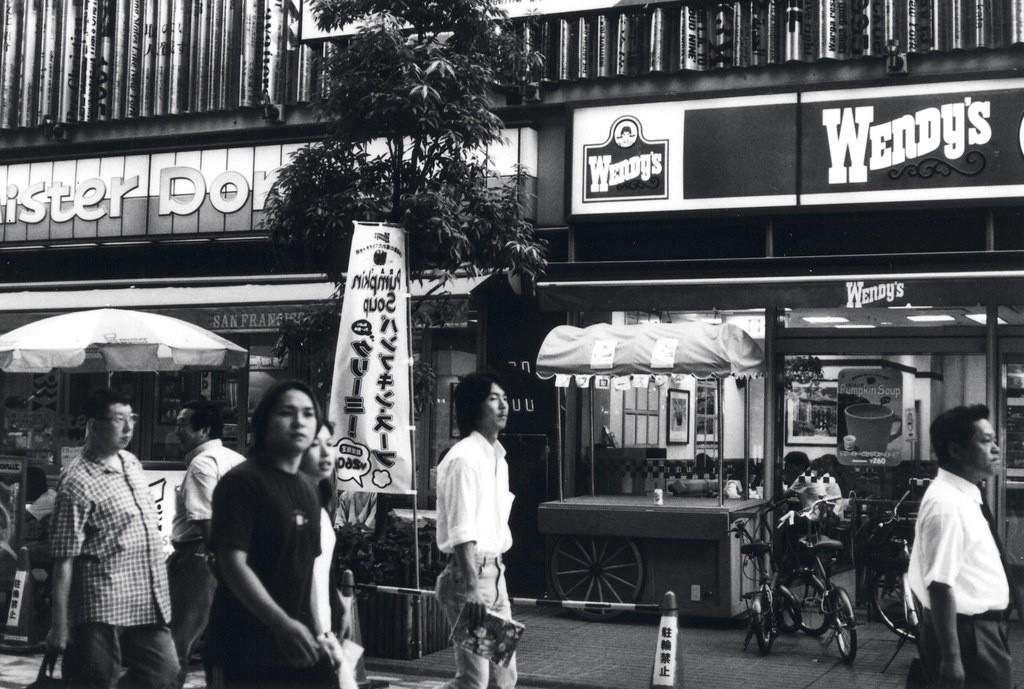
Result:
[784,397,838,447]
[696,377,721,445]
[666,387,690,445]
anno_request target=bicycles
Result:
[777,495,858,663]
[854,490,924,673]
[725,493,803,656]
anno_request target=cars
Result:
[222,411,240,438]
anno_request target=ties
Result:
[980,488,1015,609]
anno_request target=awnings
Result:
[535,323,765,392]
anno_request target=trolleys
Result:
[537,321,774,623]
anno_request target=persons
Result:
[200,379,336,689]
[783,451,843,523]
[907,404,1012,689]
[47,391,181,689]
[435,371,517,689]
[163,400,247,689]
[333,490,377,534]
[723,461,756,498]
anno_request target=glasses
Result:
[100,413,137,425]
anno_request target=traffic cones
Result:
[0,546,46,655]
[650,591,684,689]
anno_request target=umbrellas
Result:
[0,307,247,387]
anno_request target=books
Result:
[449,604,525,668]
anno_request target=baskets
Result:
[854,515,897,571]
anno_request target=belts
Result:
[979,610,1011,622]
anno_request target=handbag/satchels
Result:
[27,647,73,689]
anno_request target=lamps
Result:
[263,96,286,123]
[884,39,909,76]
[42,114,72,144]
[505,78,541,105]
